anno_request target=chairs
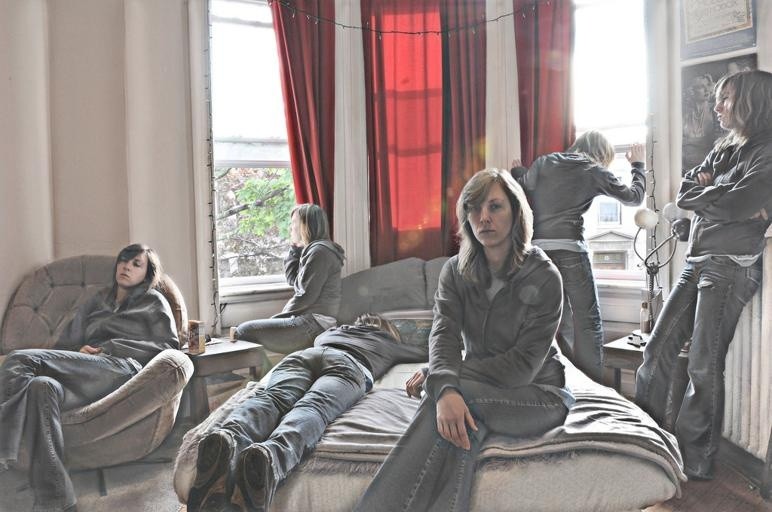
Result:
[1,257,193,499]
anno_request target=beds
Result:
[172,255,686,510]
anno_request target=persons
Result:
[0,243,179,512]
[232,203,346,383]
[187,313,429,511]
[355,167,575,511]
[512,132,644,386]
[633,70,772,480]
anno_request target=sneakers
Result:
[229,443,273,512]
[186,430,233,512]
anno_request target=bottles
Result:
[640,301,650,333]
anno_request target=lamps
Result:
[626,200,694,346]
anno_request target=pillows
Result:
[336,254,452,325]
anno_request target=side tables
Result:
[182,336,264,426]
[601,330,693,423]
